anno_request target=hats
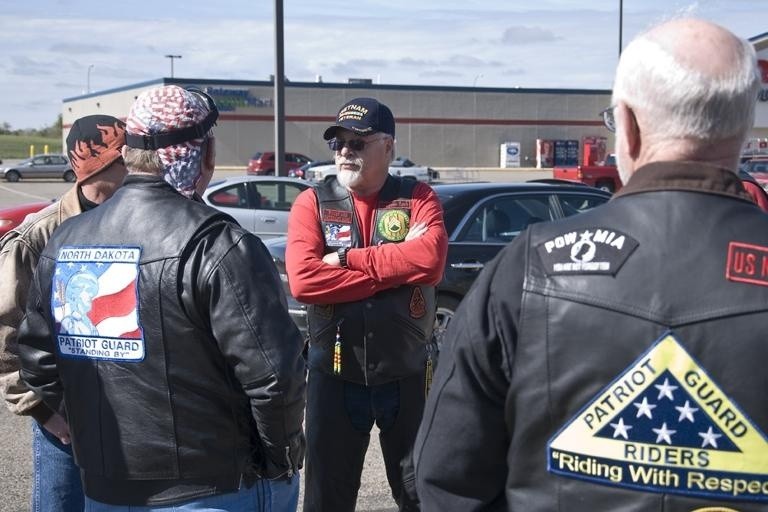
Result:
[65,114,127,184]
[323,97,395,141]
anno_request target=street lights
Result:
[88,64,95,93]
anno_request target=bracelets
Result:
[339,245,349,268]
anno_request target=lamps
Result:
[486,209,513,244]
[525,217,544,230]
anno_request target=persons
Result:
[541,142,554,168]
[13,82,308,512]
[0,114,130,511]
[58,268,101,336]
[411,17,768,512]
[285,96,449,512]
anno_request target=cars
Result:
[0,153,76,181]
[0,202,58,238]
[259,179,617,357]
[247,151,439,182]
[740,159,768,194]
[201,175,321,240]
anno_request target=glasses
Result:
[597,104,623,132]
[124,87,220,151]
[324,137,389,152]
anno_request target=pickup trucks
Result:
[552,153,624,195]
